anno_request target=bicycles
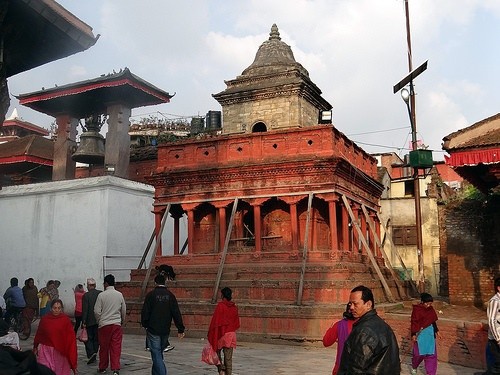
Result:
[2,301,32,340]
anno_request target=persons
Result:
[22,278,39,336]
[74,284,86,334]
[37,280,60,317]
[410,293,438,375]
[208,287,240,375]
[337,286,400,375]
[323,302,360,375]
[486,278,500,375]
[3,278,26,329]
[141,275,185,375]
[34,299,79,375]
[82,279,103,364]
[94,274,126,375]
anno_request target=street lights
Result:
[400,87,425,294]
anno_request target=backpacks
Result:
[156,265,176,278]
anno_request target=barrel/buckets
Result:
[206,111,221,129]
[191,119,204,129]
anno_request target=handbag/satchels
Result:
[78,328,88,342]
[14,318,23,333]
[201,345,221,365]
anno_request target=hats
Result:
[221,287,232,297]
[87,278,96,285]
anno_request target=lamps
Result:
[319,110,332,124]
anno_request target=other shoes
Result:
[86,353,97,365]
[410,367,417,375]
[97,368,107,374]
[164,345,174,352]
[145,347,151,352]
[113,370,120,375]
